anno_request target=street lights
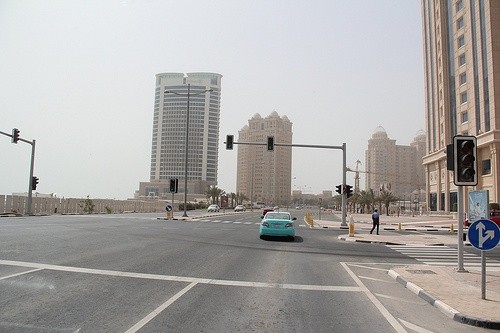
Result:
[163,82,213,218]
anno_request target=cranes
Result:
[347,160,383,195]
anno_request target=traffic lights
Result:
[347,185,353,198]
[336,185,341,194]
[454,136,478,186]
[268,138,273,150]
[226,135,233,150]
[170,178,176,193]
[13,128,20,143]
[32,177,39,190]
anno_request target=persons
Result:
[370,208,380,234]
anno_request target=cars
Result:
[262,209,274,217]
[464,208,500,228]
[259,211,297,241]
[207,204,220,213]
[234,205,246,212]
